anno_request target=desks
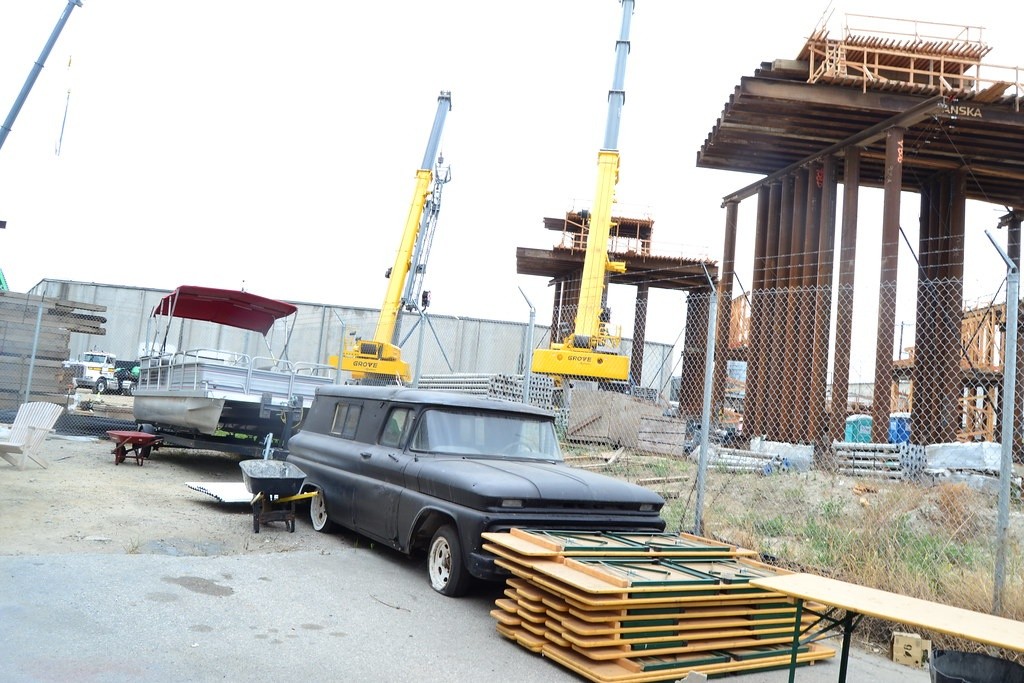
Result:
[481,528,836,683]
[748,572,1024,683]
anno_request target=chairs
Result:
[0,402,64,472]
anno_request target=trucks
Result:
[67,350,137,396]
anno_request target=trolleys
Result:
[105,430,163,466]
[238,458,319,533]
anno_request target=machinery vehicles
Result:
[525,1,636,407]
[325,88,455,384]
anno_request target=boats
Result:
[132,285,305,438]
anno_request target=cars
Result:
[663,400,680,417]
[284,383,667,598]
